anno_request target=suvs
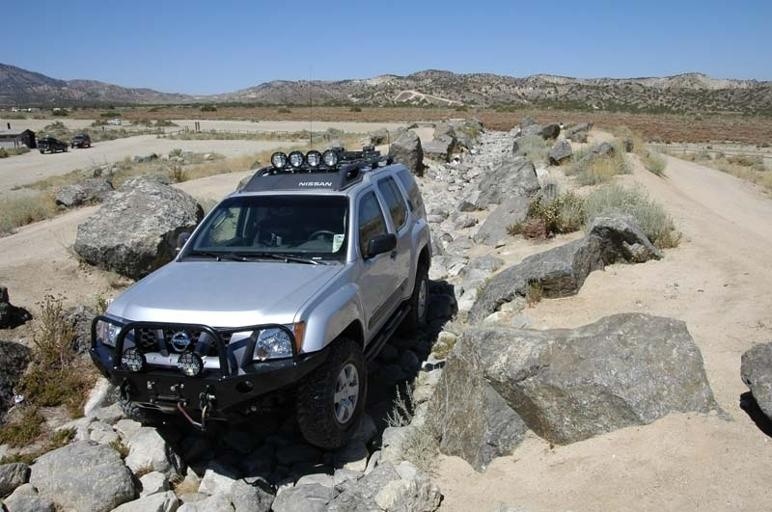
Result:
[70,133,90,148]
[37,138,67,153]
[90,147,432,448]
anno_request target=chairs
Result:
[252,203,310,249]
